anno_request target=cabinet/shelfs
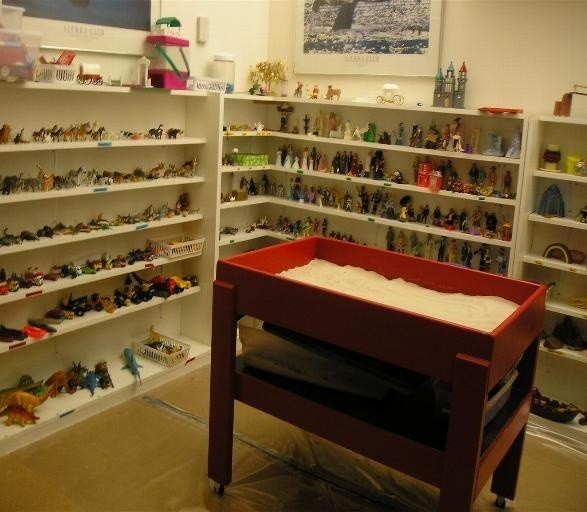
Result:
[509,113,587,443]
[215,94,530,277]
[0,81,222,456]
[206,235,548,512]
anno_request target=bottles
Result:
[214,53,235,91]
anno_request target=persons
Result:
[220,144,513,201]
[217,173,512,240]
[388,227,508,275]
